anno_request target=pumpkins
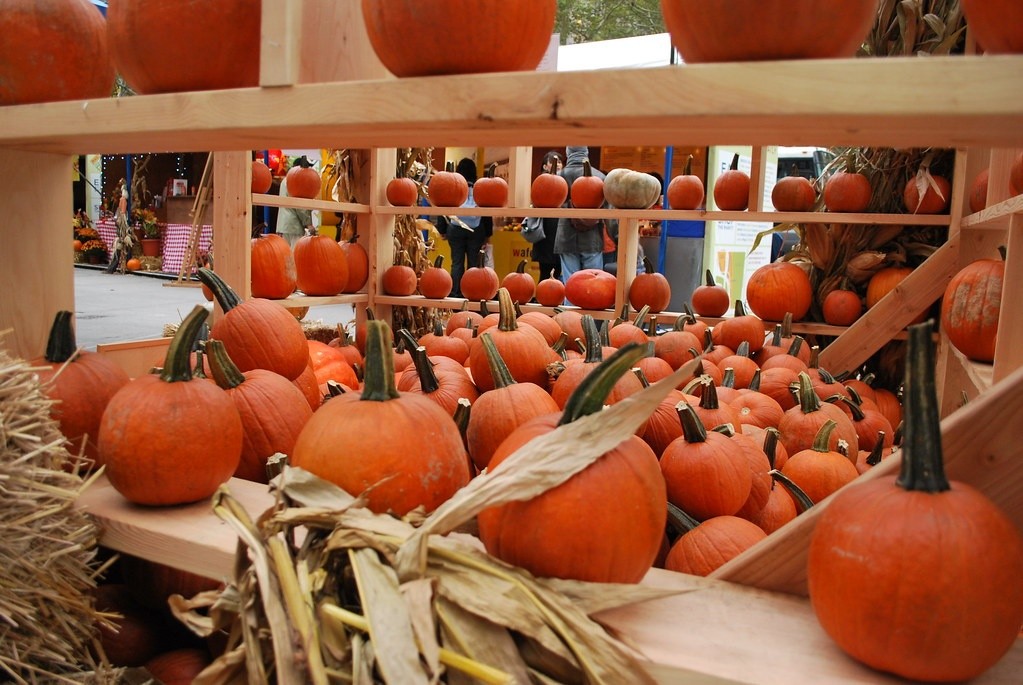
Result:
[0,0,1023,685]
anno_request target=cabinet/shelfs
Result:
[0,0,1023,685]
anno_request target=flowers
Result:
[79,228,106,252]
[136,209,159,239]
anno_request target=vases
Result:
[89,252,100,264]
[134,229,160,257]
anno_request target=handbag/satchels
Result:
[601,219,616,253]
[520,217,546,243]
[482,243,494,270]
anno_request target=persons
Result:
[276,157,316,249]
[531,144,607,307]
[437,157,493,298]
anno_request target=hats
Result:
[566,146,588,157]
[293,158,314,168]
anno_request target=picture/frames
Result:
[173,179,187,196]
